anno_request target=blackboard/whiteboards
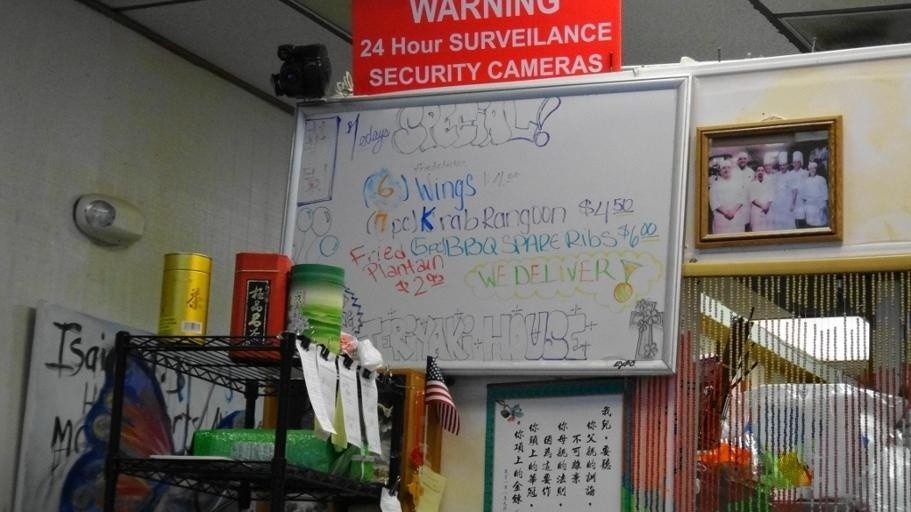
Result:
[13,299,265,512]
[279,76,693,377]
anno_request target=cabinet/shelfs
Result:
[100,328,406,512]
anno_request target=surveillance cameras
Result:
[272,44,331,98]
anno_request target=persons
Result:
[705,142,829,234]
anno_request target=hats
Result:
[763,152,772,165]
[720,160,731,169]
[778,152,787,165]
[793,151,801,162]
[737,152,747,160]
[808,162,817,169]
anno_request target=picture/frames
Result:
[692,114,846,252]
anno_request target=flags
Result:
[423,354,460,436]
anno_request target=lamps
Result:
[74,192,147,249]
